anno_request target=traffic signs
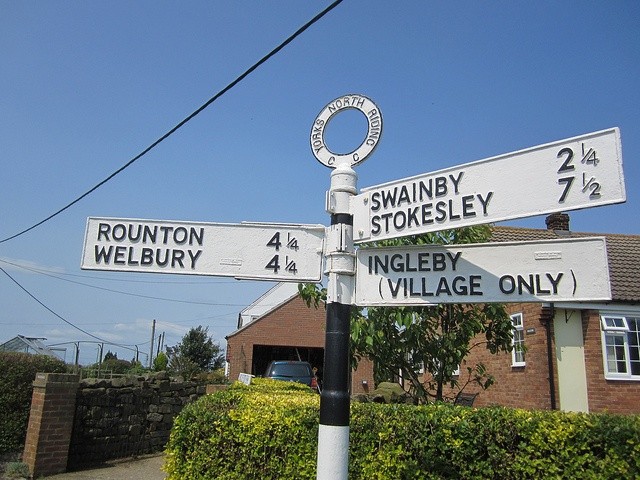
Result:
[349,126,628,242]
[351,235,613,307]
[78,215,325,283]
